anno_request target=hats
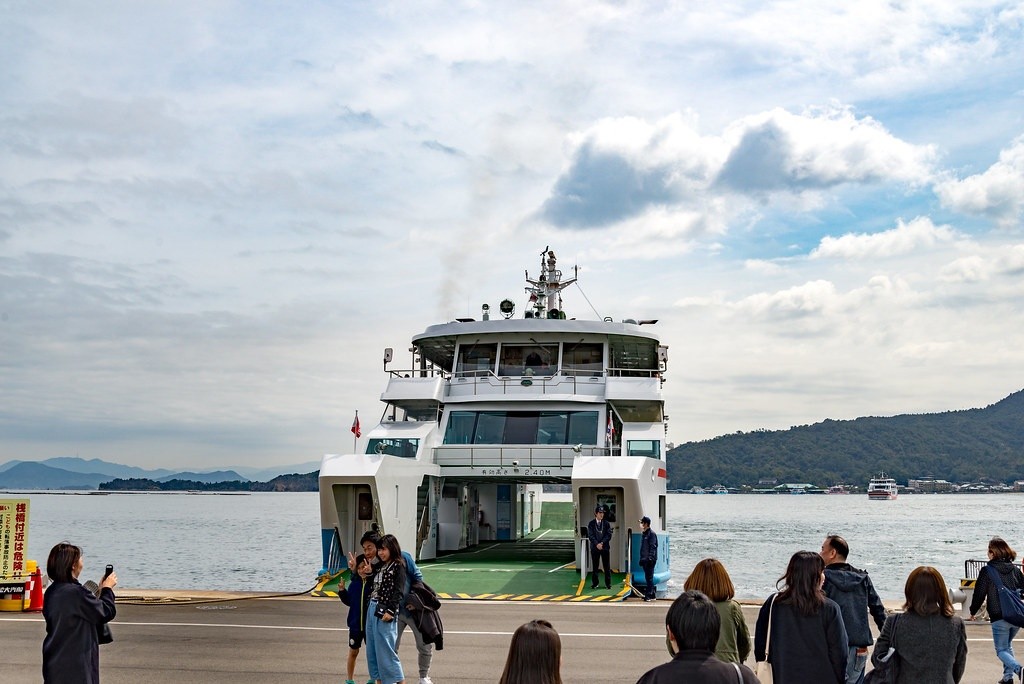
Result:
[596,507,606,513]
[639,516,650,525]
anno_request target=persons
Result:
[871,566,968,684]
[348,530,434,684]
[640,516,658,601]
[968,537,1024,684]
[588,506,612,589]
[498,618,562,684]
[754,551,849,684]
[42,540,118,684]
[820,535,887,684]
[684,558,751,665]
[337,555,375,684]
[362,536,406,684]
[636,590,761,684]
[507,363,549,376]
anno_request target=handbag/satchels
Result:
[374,604,394,623]
[862,613,901,684]
[986,565,1024,629]
[753,661,773,684]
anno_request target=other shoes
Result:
[1019,667,1024,684]
[605,585,611,589]
[591,584,598,589]
[419,677,433,684]
[998,679,1013,684]
[344,679,355,684]
[366,678,375,684]
[644,596,656,602]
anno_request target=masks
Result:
[640,524,644,529]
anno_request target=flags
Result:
[351,417,361,438]
[607,418,615,439]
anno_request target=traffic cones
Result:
[23,569,44,612]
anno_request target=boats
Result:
[867,469,899,501]
[306,241,674,602]
[948,557,1024,626]
[706,483,729,495]
[689,485,707,494]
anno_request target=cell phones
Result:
[105,565,113,579]
